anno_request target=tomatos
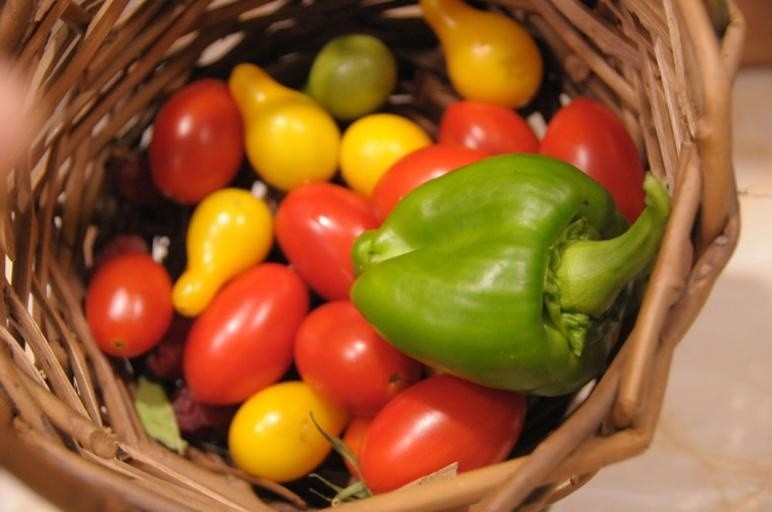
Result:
[85,1,646,495]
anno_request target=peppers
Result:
[349,148,668,396]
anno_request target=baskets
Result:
[0,0,748,512]
